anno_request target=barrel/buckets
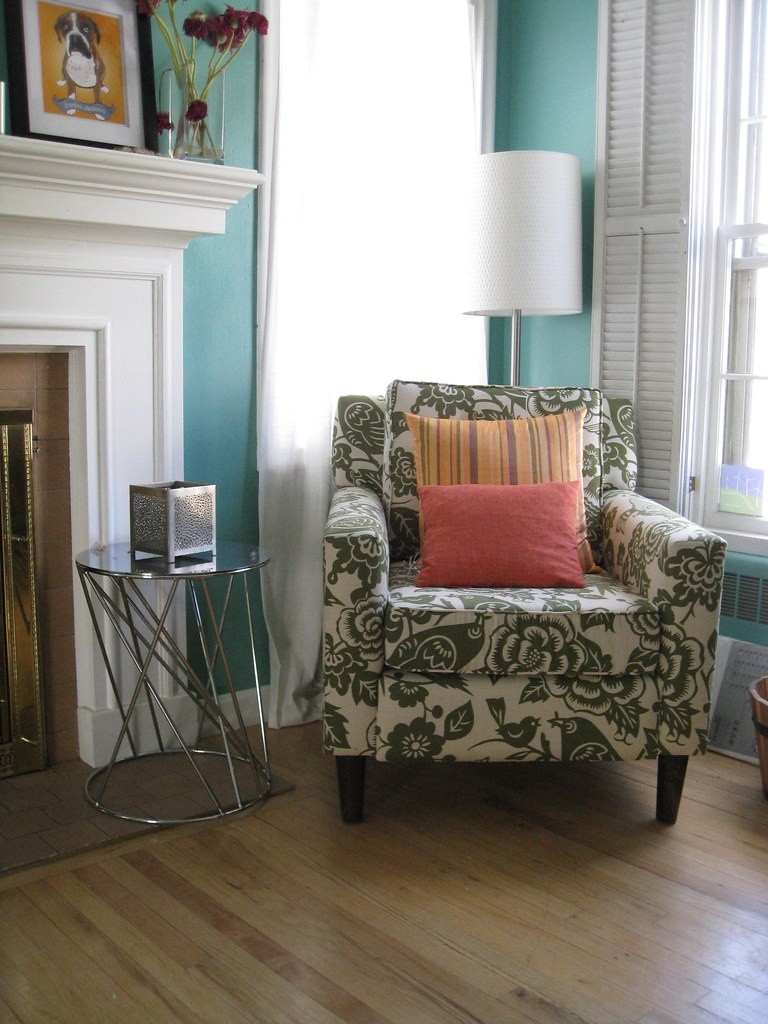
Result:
[748,675,768,803]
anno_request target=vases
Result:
[168,63,227,164]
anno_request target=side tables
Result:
[74,540,275,826]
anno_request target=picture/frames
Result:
[4,0,160,155]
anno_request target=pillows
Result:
[414,481,586,588]
[407,412,604,573]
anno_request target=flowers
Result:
[132,0,270,135]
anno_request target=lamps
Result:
[459,152,586,387]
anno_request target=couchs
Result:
[322,379,729,829]
